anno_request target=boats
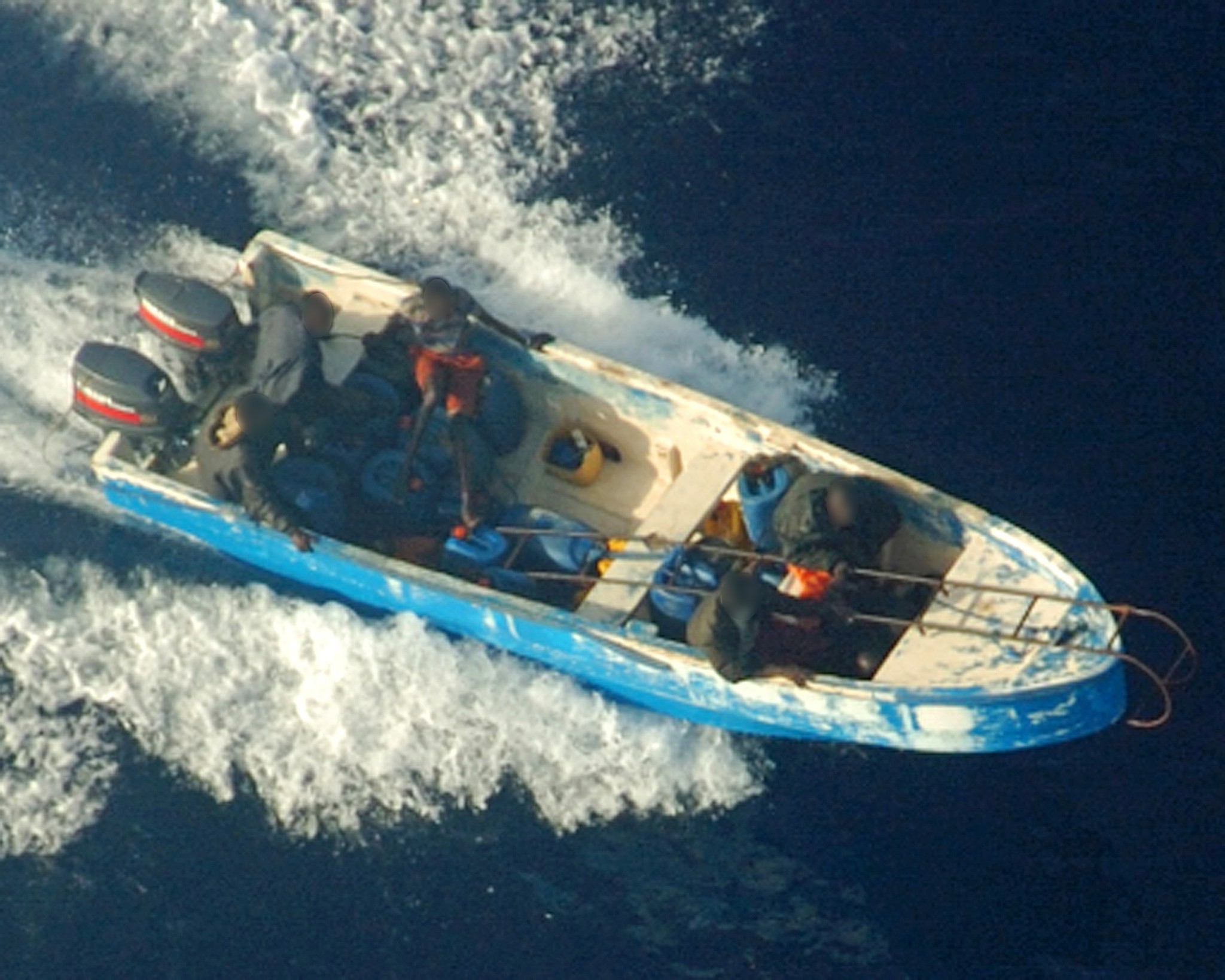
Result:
[72,229,1200,756]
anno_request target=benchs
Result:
[493,418,671,520]
[574,437,752,625]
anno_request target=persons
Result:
[392,277,555,529]
[772,471,902,585]
[256,290,335,417]
[685,570,841,689]
[194,392,312,552]
